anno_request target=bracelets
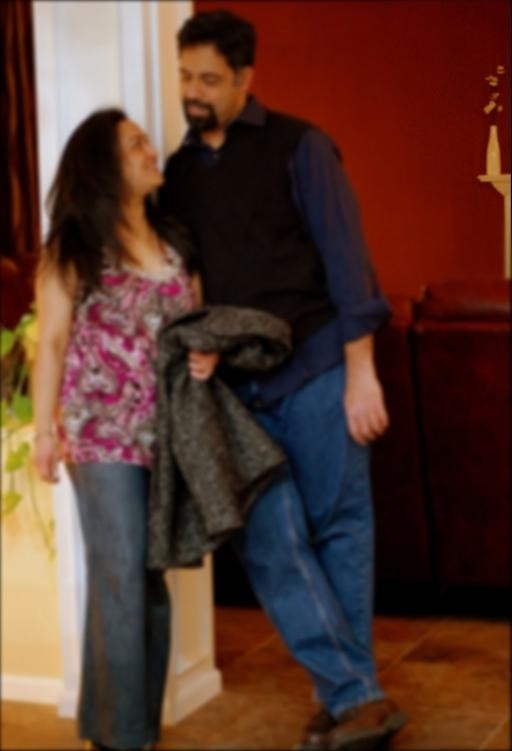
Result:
[33,429,51,443]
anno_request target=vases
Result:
[485,125,501,175]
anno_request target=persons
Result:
[30,105,221,750]
[146,10,413,748]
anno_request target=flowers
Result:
[479,63,507,124]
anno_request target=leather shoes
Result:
[299,695,410,750]
